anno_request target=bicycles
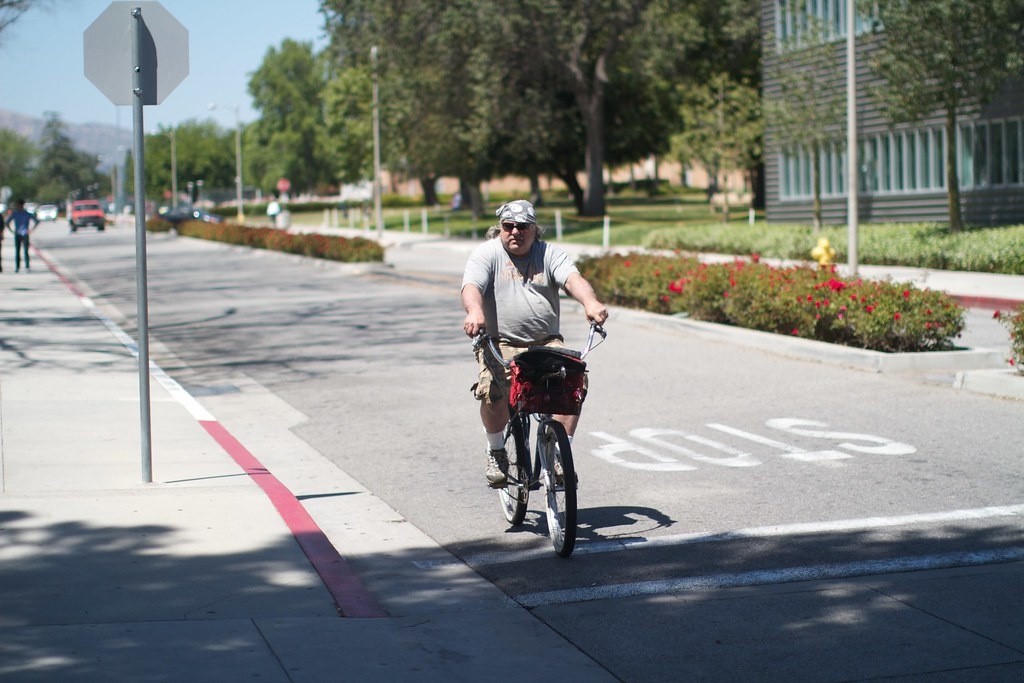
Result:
[477,320,607,557]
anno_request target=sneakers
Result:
[553,454,578,487]
[485,447,509,484]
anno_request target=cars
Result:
[24,203,59,221]
[68,199,106,231]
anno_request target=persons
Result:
[459,200,609,487]
[5,198,41,273]
[0,213,5,273]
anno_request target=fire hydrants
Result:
[811,236,835,269]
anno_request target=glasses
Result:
[502,222,532,231]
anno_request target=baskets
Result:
[513,350,586,415]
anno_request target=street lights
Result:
[207,86,242,223]
